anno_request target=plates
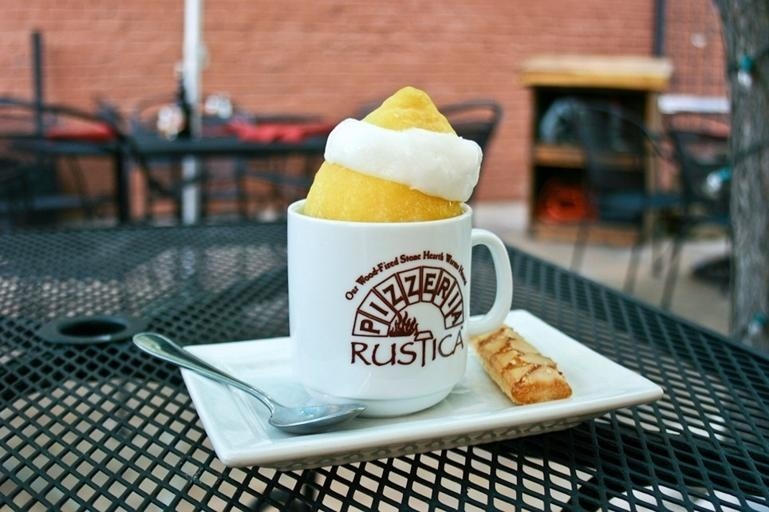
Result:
[177,308,665,471]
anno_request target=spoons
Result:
[132,332,364,435]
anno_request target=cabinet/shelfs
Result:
[516,50,675,245]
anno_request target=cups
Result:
[286,200,514,417]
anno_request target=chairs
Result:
[0,94,503,435]
[563,104,730,311]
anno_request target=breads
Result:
[475,323,573,405]
